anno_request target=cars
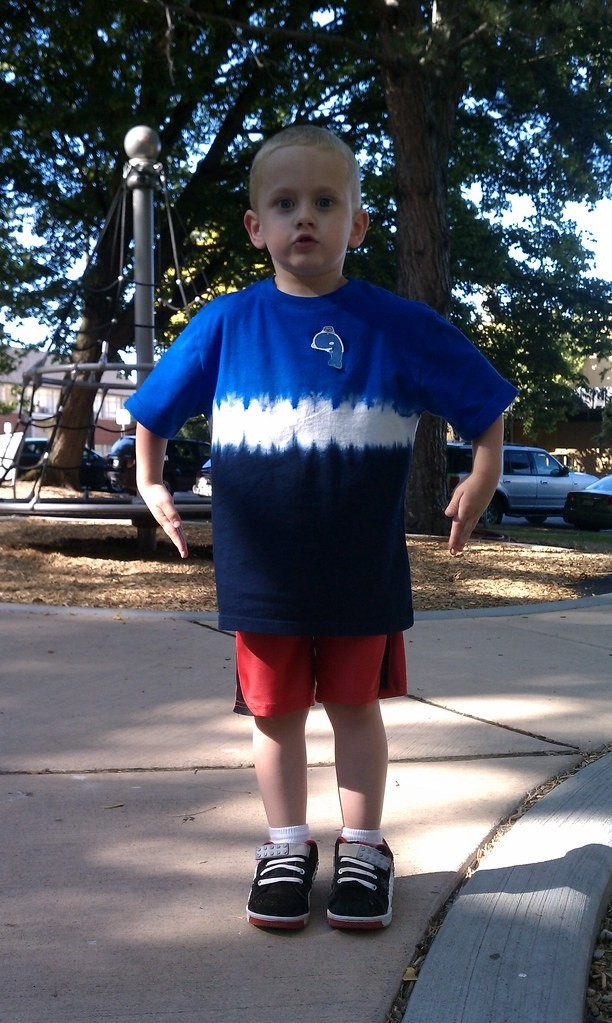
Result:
[191,455,211,497]
[562,473,612,533]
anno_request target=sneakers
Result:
[245,840,319,928]
[327,836,395,930]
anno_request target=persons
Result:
[120,123,519,930]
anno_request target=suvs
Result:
[17,437,107,491]
[446,441,600,527]
[104,435,212,498]
